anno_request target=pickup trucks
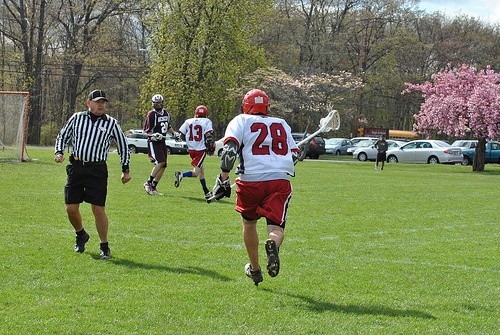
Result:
[461,141,500,166]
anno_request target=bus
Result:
[357,128,418,140]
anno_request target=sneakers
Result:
[153,189,163,196]
[245,263,263,283]
[175,171,183,188]
[74,232,89,253]
[99,248,110,259]
[144,182,152,195]
[205,192,214,203]
[264,239,280,277]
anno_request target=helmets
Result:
[152,94,164,106]
[242,89,269,114]
[195,105,208,116]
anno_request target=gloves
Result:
[172,132,180,142]
[151,132,163,141]
[213,173,231,200]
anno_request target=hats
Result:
[89,90,108,102]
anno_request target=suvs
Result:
[291,133,326,160]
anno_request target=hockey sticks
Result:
[206,109,342,205]
[124,129,176,140]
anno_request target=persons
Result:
[213,89,299,283]
[371,134,388,170]
[174,105,213,199]
[54,90,132,260]
[143,94,179,195]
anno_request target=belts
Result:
[74,161,104,166]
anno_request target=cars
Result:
[214,137,225,157]
[451,140,480,151]
[353,140,413,161]
[350,137,378,143]
[386,140,463,165]
[324,138,355,156]
[125,133,188,155]
[127,130,147,135]
[347,140,376,152]
